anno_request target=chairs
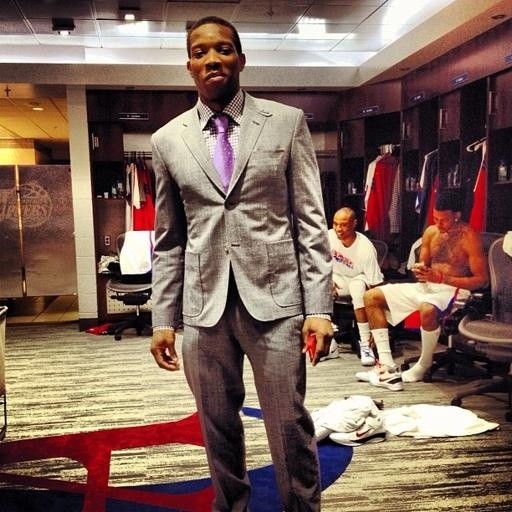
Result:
[101,229,161,340]
[336,238,511,427]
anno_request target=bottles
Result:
[498,159,508,181]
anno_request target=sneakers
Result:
[358,339,376,366]
[319,343,339,362]
[309,394,386,448]
[355,363,404,392]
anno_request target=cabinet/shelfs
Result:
[341,67,512,260]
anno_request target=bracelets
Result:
[438,272,443,284]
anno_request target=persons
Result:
[355,190,488,383]
[150,16,334,512]
[319,207,384,366]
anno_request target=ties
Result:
[211,114,234,195]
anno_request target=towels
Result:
[118,229,154,275]
